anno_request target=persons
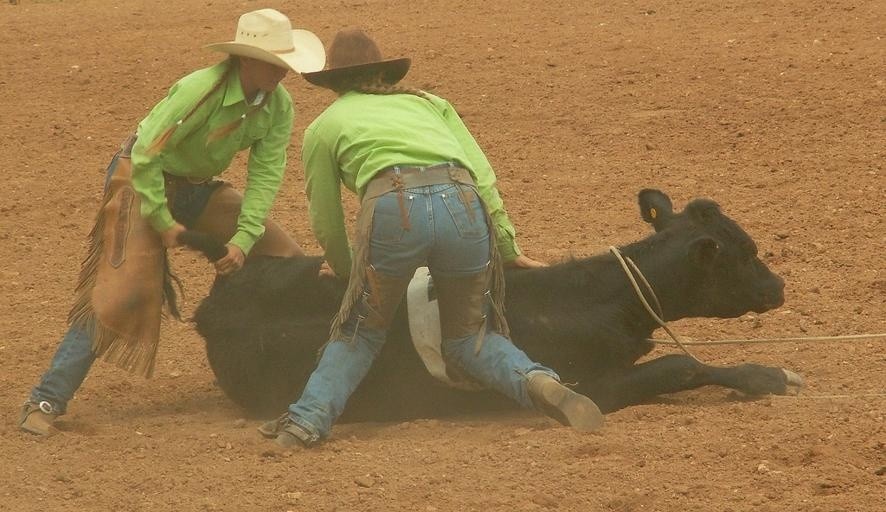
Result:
[254,27,608,447]
[18,6,326,440]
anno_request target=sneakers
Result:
[528,373,604,436]
[261,419,306,457]
[18,400,58,438]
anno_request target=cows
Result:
[161,189,806,427]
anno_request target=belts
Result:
[378,162,459,175]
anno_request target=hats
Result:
[206,8,326,76]
[302,29,410,85]
